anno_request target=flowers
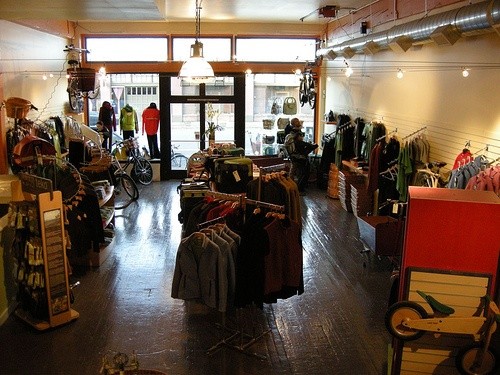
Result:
[202,102,223,139]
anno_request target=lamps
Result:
[178,0,214,79]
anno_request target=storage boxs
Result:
[327,163,404,256]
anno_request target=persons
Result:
[284,117,318,196]
[94,122,110,148]
[141,103,161,155]
[98,101,116,153]
[120,104,139,156]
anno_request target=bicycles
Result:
[0,97,38,175]
[104,136,154,210]
[63,43,100,110]
[171,145,188,169]
[385,290,500,375]
[299,68,317,109]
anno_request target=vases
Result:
[209,131,215,146]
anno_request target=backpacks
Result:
[284,134,297,158]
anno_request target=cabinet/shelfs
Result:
[71,185,115,267]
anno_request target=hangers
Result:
[181,142,291,244]
[79,145,112,173]
[58,163,84,212]
